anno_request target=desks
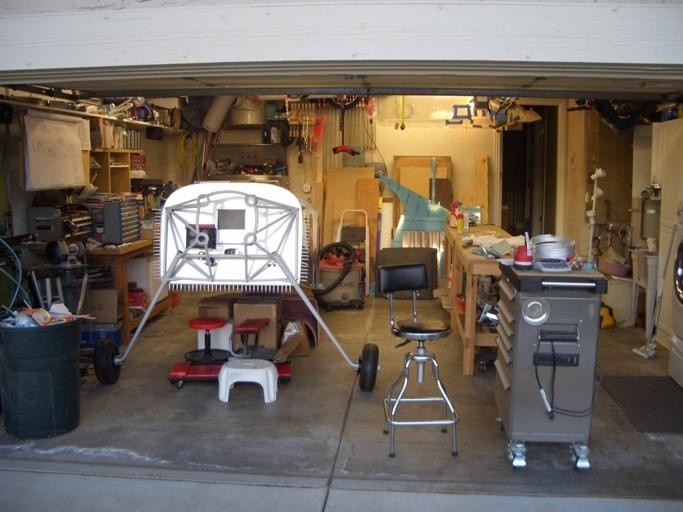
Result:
[51,234,152,347]
[444,223,535,377]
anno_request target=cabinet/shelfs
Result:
[84,145,145,207]
[628,116,682,353]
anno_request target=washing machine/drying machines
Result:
[668,205,683,390]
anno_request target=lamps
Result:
[585,168,608,231]
[442,95,543,131]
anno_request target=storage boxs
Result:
[231,299,284,353]
[86,288,117,324]
[197,294,231,322]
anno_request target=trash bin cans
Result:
[0,317,84,438]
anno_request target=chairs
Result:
[373,265,458,462]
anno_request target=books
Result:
[63,119,147,247]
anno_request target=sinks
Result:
[628,246,658,290]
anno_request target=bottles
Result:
[447,201,469,233]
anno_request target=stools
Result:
[215,355,281,405]
[184,316,230,366]
[233,316,270,355]
